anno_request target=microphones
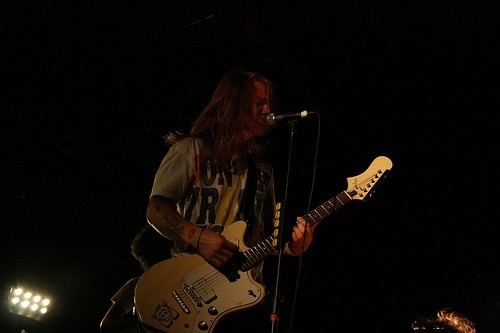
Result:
[265,110,307,126]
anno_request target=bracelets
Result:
[196,228,205,255]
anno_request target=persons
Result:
[98,225,175,333]
[147,68,315,333]
[410,307,477,332]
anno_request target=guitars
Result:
[135,155,393,333]
